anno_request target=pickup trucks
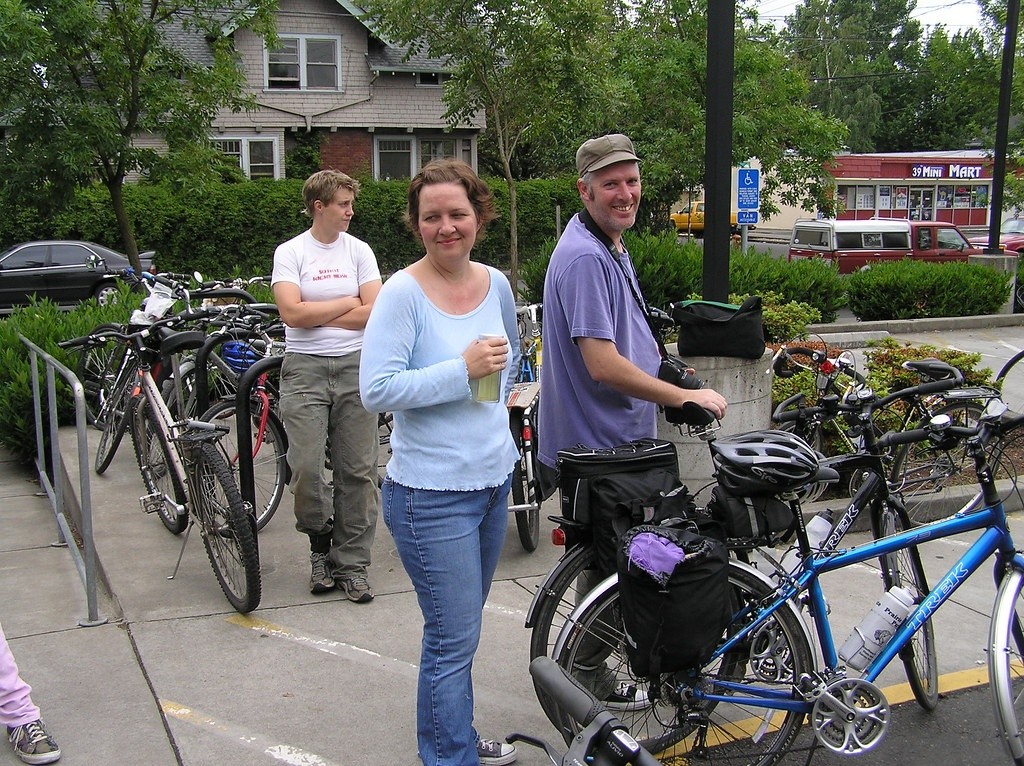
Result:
[788,216,984,278]
[669,201,739,240]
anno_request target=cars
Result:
[0,241,156,314]
[965,215,1024,254]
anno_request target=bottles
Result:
[470,332,505,404]
[838,584,916,670]
[776,508,835,579]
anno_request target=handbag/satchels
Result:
[673,295,765,360]
[557,437,793,678]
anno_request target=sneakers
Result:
[475,738,517,766]
[308,551,337,593]
[6,716,61,764]
[599,680,651,710]
[339,576,374,602]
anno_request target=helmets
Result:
[222,340,259,376]
[711,429,820,488]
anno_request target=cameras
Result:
[658,359,703,391]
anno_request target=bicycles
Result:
[503,330,1023,765]
[508,302,542,554]
[58,255,395,615]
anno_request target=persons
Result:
[270,168,382,603]
[357,161,523,766]
[537,134,728,711]
[0,624,62,765]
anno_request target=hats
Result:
[575,134,643,178]
